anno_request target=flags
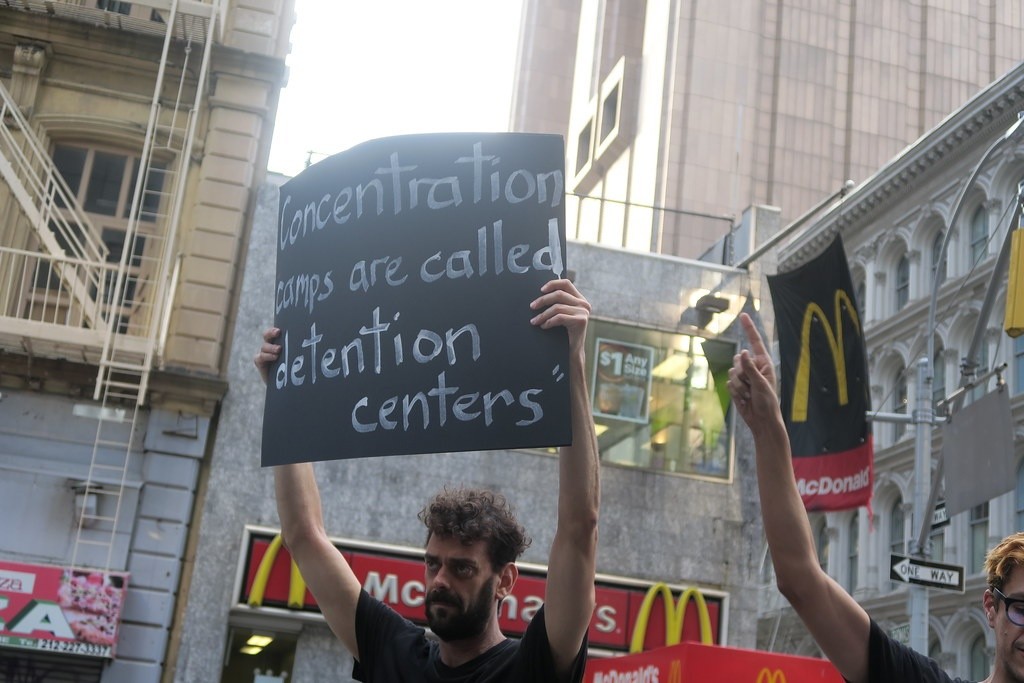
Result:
[768,233,875,512]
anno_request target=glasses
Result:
[993,587,1024,626]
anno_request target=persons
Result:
[252,279,601,683]
[726,312,1024,683]
